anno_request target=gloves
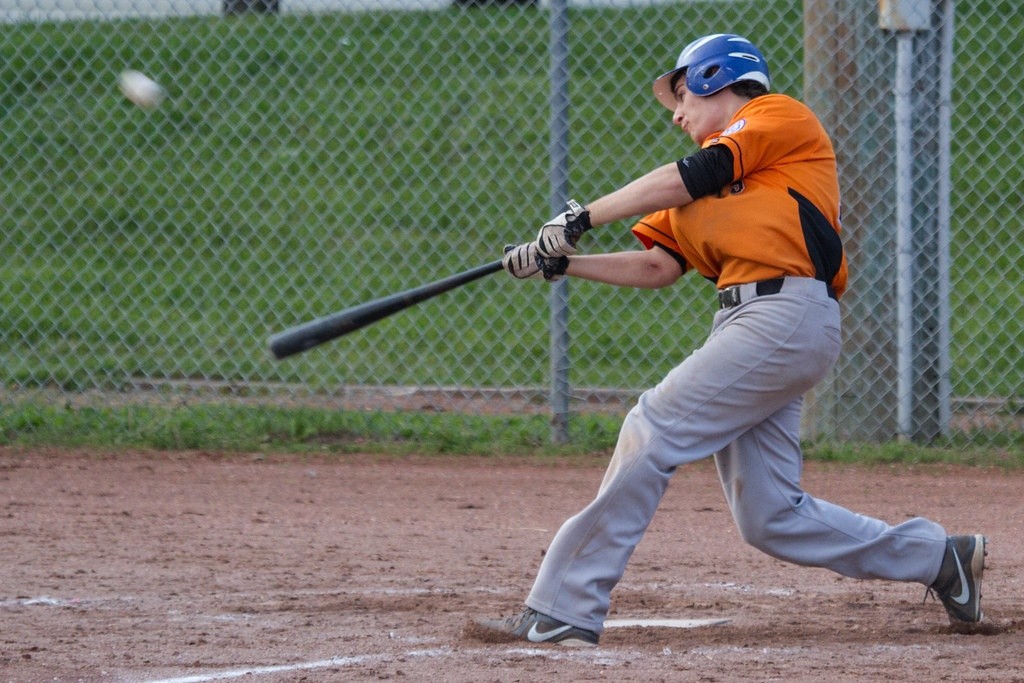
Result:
[536,197,593,258]
[502,242,571,281]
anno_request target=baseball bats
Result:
[263,258,504,362]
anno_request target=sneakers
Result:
[921,534,989,624]
[471,608,599,649]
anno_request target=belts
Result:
[718,277,839,310]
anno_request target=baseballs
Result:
[116,68,168,112]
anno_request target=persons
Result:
[471,34,988,648]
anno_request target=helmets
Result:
[653,34,770,112]
